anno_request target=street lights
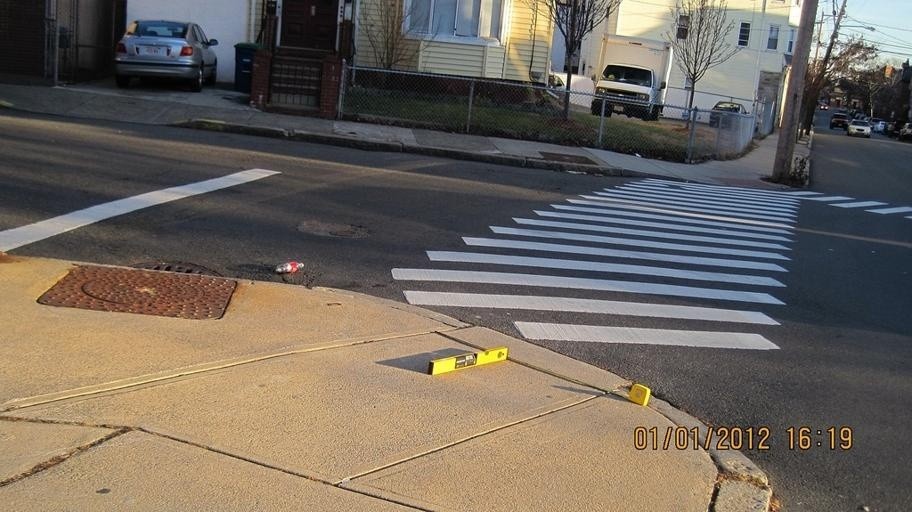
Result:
[837,25,876,32]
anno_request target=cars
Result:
[820,104,828,110]
[112,19,219,90]
[709,100,750,128]
[848,119,872,138]
[855,113,912,141]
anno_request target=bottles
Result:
[276,262,304,274]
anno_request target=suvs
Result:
[830,112,848,131]
[590,62,666,122]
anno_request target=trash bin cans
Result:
[234,43,257,93]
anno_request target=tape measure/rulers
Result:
[629,384,651,406]
